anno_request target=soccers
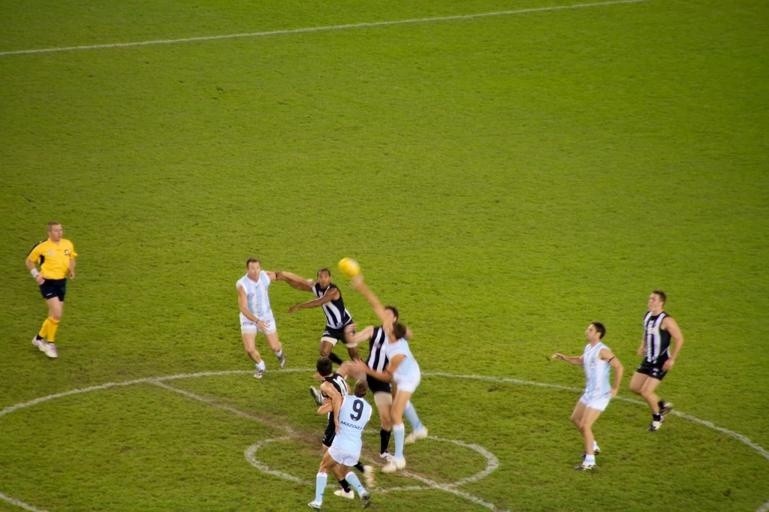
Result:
[338,258,361,278]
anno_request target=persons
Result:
[551,321,625,469]
[25,219,79,358]
[308,377,372,510]
[235,257,315,380]
[316,357,376,499]
[350,273,429,474]
[345,305,415,461]
[274,268,367,398]
[626,290,685,432]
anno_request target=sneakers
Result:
[311,387,323,404]
[584,449,600,458]
[650,416,664,431]
[278,353,285,368]
[379,428,428,473]
[310,372,326,381]
[308,465,377,509]
[660,402,674,415]
[574,465,598,473]
[45,345,58,358]
[254,367,265,379]
[32,336,48,351]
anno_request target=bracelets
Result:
[30,268,40,278]
[255,318,260,324]
